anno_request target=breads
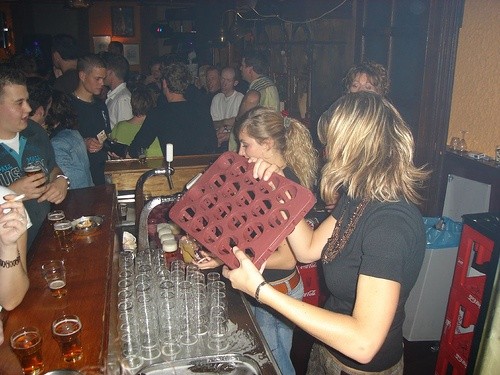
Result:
[157,219,180,252]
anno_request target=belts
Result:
[270,267,300,294]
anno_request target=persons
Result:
[339,59,390,96]
[213,90,428,375]
[189,106,324,374]
[0,28,389,358]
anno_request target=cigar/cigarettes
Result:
[11,194,26,201]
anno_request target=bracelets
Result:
[0,250,20,268]
[224,125,227,129]
[56,174,71,190]
[255,281,267,305]
[224,129,228,133]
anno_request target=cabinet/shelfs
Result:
[435,223,495,375]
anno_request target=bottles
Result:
[433,217,446,231]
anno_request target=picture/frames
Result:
[111,6,135,37]
[92,35,111,54]
[122,43,140,65]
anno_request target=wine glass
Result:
[456,130,470,152]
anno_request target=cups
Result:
[41,260,68,298]
[9,326,45,375]
[48,210,66,237]
[52,313,84,363]
[23,161,44,189]
[118,234,230,372]
[449,137,460,150]
[53,220,73,249]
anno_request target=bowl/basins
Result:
[71,216,103,236]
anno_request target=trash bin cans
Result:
[402,215,476,343]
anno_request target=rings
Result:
[94,150,96,153]
